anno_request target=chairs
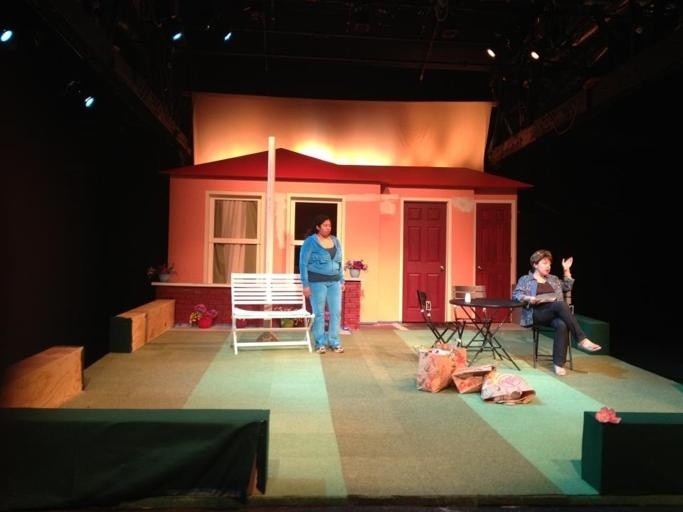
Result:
[416,289,470,363]
[452,285,499,359]
[532,304,574,371]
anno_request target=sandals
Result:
[554,364,566,376]
[578,340,601,352]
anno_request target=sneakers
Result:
[333,346,344,353]
[317,347,325,354]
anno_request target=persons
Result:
[297,214,346,356]
[510,249,603,377]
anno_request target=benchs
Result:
[230,271,316,355]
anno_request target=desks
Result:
[447,296,526,371]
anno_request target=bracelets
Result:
[563,267,570,272]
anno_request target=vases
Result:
[350,268,361,279]
[157,272,170,283]
[197,318,213,329]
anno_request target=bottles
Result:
[464,293,470,303]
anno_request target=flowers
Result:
[146,260,178,276]
[189,304,218,324]
[343,259,369,274]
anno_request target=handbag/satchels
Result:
[417,342,536,404]
